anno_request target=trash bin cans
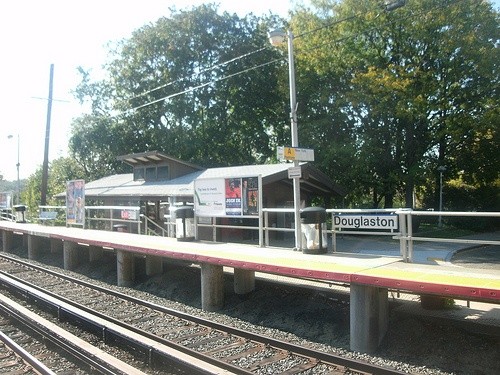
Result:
[297,205,330,255]
[13,203,29,223]
[172,205,199,244]
